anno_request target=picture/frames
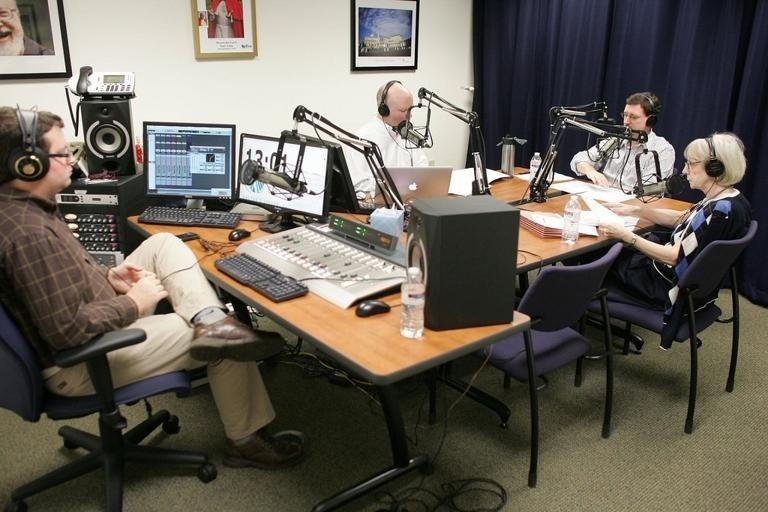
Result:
[191,0,258,61]
[0,0,72,80]
[350,0,420,71]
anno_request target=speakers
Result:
[406,196,520,332]
[79,98,137,176]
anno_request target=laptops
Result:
[283,129,374,214]
[374,166,454,209]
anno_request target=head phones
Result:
[378,80,401,116]
[704,135,724,178]
[8,105,50,181]
[646,96,658,128]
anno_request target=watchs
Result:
[629,234,639,247]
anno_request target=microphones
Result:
[240,159,307,195]
[635,174,684,196]
[399,120,427,146]
[599,137,618,157]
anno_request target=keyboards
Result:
[217,253,308,303]
[138,205,240,228]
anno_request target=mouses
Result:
[229,229,251,241]
[356,300,390,316]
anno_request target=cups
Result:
[546,162,553,183]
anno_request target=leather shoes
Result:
[190,316,284,361]
[222,432,303,467]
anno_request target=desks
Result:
[517,193,700,385]
[125,198,532,512]
[330,165,561,223]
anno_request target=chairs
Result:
[0,310,218,512]
[574,220,759,434]
[427,241,624,488]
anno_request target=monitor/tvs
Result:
[237,133,333,233]
[142,120,236,209]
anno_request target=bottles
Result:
[529,153,542,185]
[501,138,515,177]
[399,267,425,339]
[562,195,581,245]
[472,152,485,194]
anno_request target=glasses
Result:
[685,161,700,169]
[48,146,73,164]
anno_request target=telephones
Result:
[76,66,135,96]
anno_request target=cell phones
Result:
[177,232,199,241]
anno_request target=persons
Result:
[341,80,430,201]
[0,0,55,56]
[199,12,207,26]
[570,91,676,198]
[578,133,753,307]
[0,104,300,470]
[208,0,235,38]
